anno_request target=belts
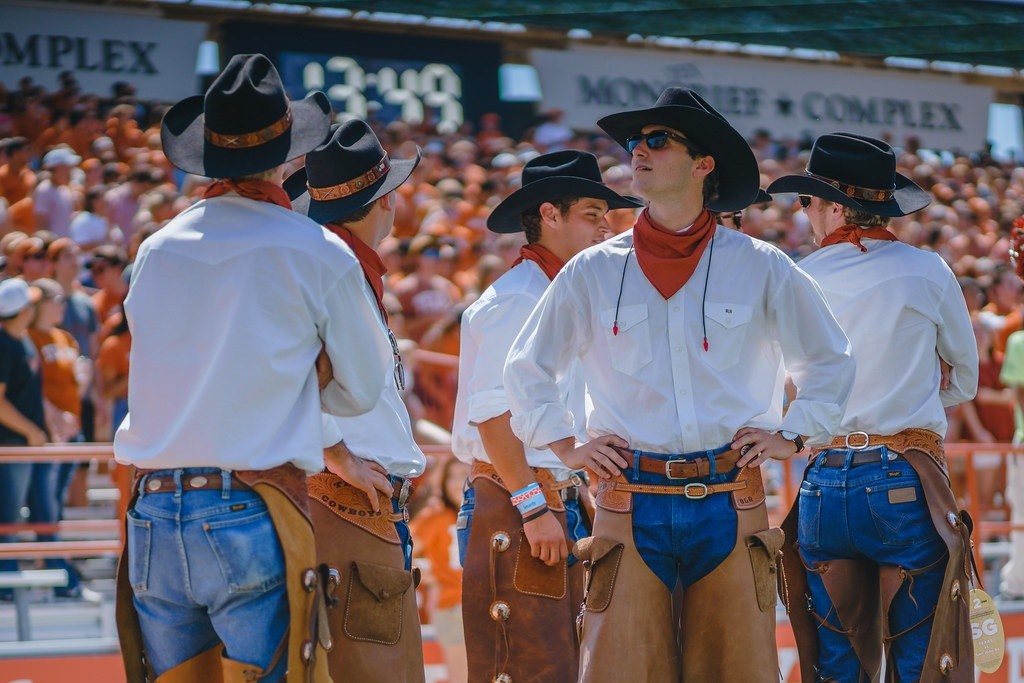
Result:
[138,472,252,494]
[824,449,905,467]
[560,486,580,503]
[386,478,416,504]
[610,443,748,478]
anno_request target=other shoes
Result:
[53,586,104,607]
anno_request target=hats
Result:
[750,188,773,205]
[0,277,43,320]
[288,118,422,224]
[68,210,108,245]
[766,132,933,218]
[595,84,759,214]
[158,53,333,178]
[484,148,644,234]
[83,242,126,270]
[41,148,82,167]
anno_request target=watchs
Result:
[776,431,806,454]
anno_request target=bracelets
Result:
[512,483,546,515]
[520,506,550,524]
[509,484,542,507]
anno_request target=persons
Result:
[305,122,429,683]
[767,130,979,683]
[443,149,644,683]
[115,55,424,683]
[505,84,858,683]
[0,71,1024,605]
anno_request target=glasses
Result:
[799,193,819,209]
[627,130,702,161]
[715,209,745,229]
